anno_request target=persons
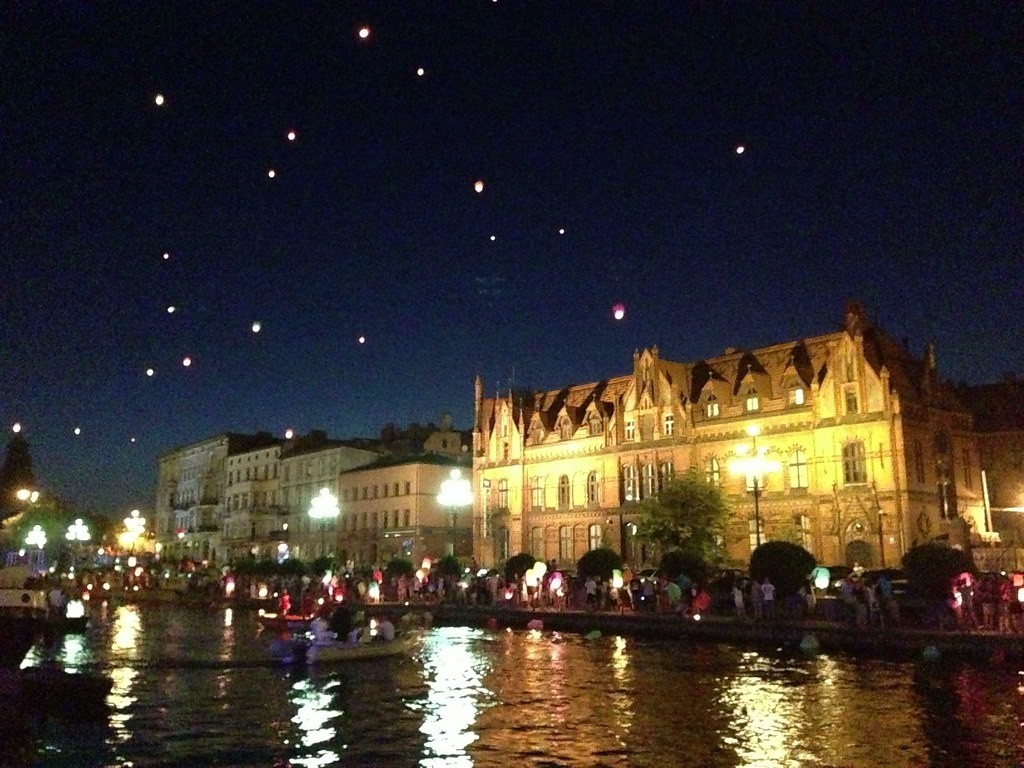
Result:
[48,559,1023,634]
[304,601,396,645]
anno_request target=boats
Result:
[306,626,418,666]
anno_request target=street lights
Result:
[727,422,782,547]
[436,468,475,560]
[308,486,339,556]
